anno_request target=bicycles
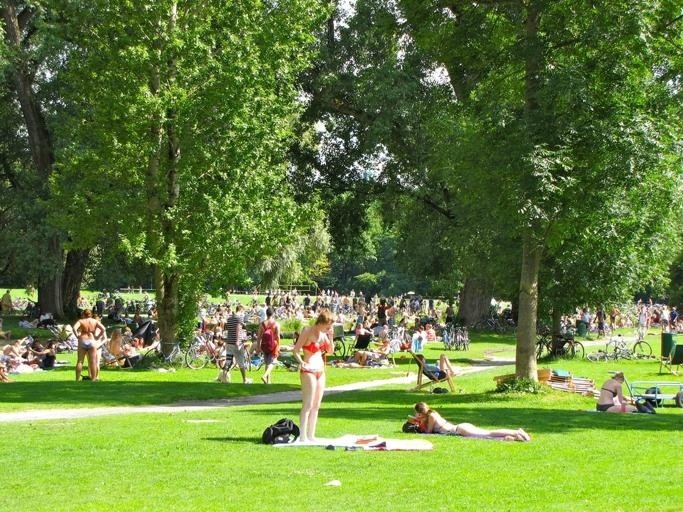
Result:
[333,324,345,357]
[473,315,515,335]
[142,334,251,371]
[442,320,468,350]
[536,330,584,359]
[586,327,652,363]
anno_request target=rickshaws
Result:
[608,371,682,408]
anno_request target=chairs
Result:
[659,331,683,377]
[405,349,457,393]
[346,333,372,362]
[98,341,148,371]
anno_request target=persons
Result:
[186,288,458,391]
[595,372,638,412]
[559,299,683,342]
[0,288,158,383]
[414,402,538,441]
[293,309,334,441]
